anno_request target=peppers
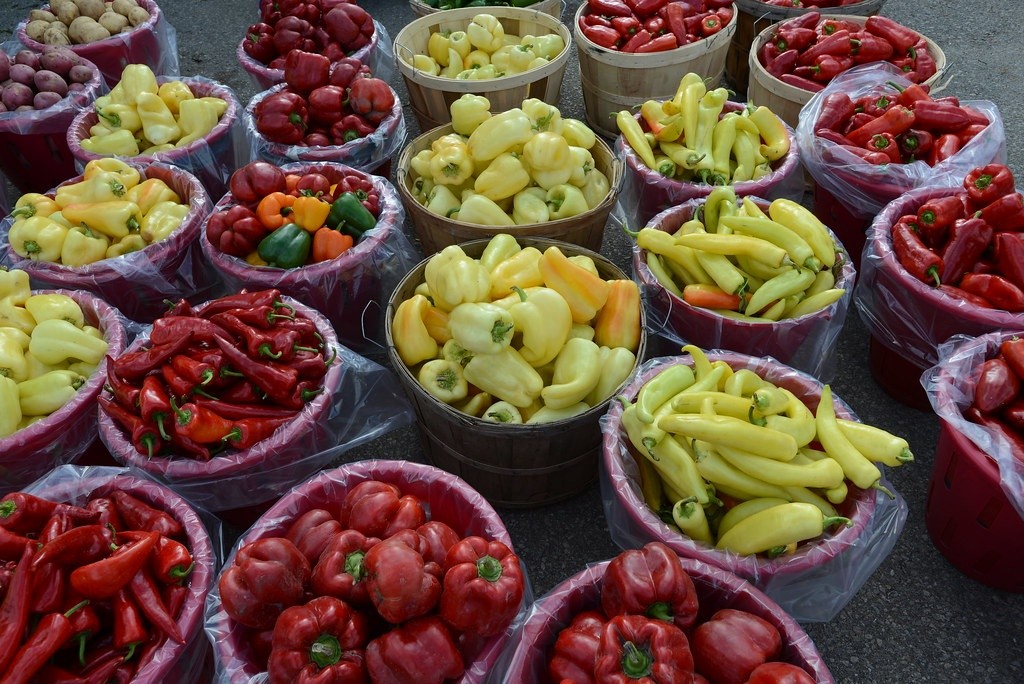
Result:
[0,2,1024,684]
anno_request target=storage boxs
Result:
[0,0,1024,684]
